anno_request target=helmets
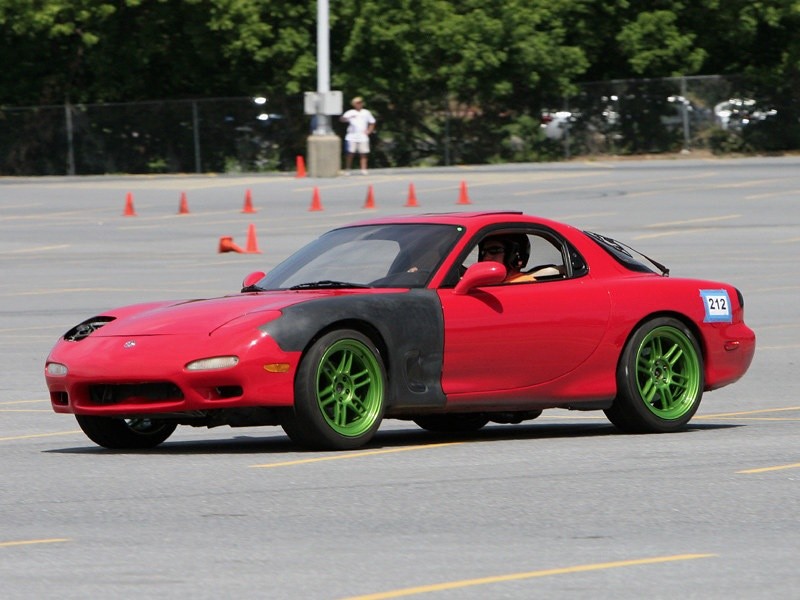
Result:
[478,233,531,268]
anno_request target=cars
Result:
[538,93,778,142]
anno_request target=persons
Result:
[478,233,536,284]
[341,98,376,175]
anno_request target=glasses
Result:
[481,245,505,257]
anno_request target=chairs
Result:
[390,247,459,285]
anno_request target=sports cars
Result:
[44,210,757,455]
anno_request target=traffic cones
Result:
[361,185,376,209]
[123,192,139,216]
[307,185,323,212]
[219,235,246,253]
[243,224,263,255]
[177,192,189,214]
[240,189,256,214]
[296,156,307,178]
[454,180,473,205]
[402,181,420,208]
